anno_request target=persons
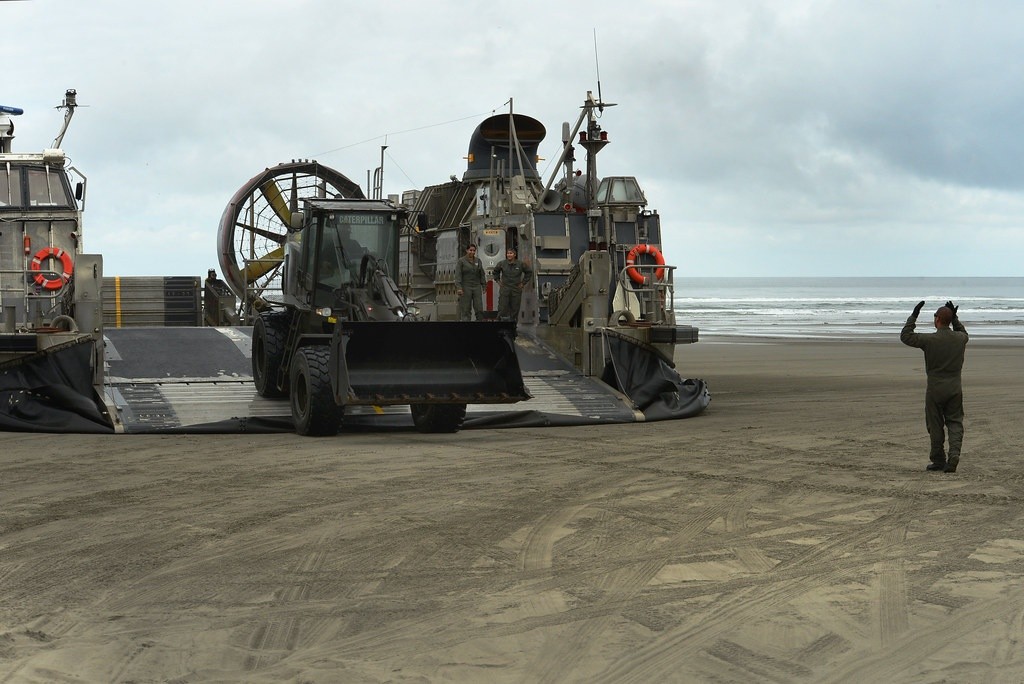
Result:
[901,301,968,472]
[492,248,531,336]
[455,244,488,321]
[321,224,363,257]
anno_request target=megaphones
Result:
[542,190,561,211]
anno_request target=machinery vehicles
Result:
[252,199,535,436]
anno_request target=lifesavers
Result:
[627,245,666,283]
[29,247,74,288]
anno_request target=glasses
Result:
[934,313,938,318]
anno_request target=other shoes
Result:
[927,463,944,471]
[942,464,957,472]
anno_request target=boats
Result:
[0,28,699,435]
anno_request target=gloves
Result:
[911,300,925,319]
[945,301,959,319]
